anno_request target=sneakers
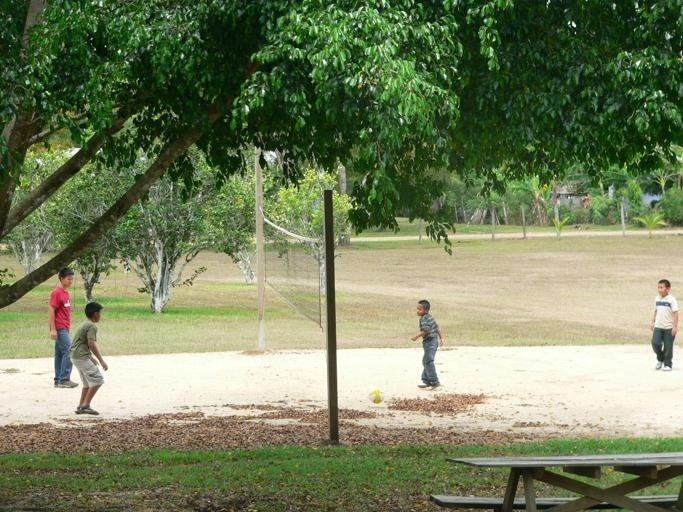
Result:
[75,407,79,414]
[417,384,429,388]
[656,361,662,369]
[79,407,98,415]
[57,380,78,388]
[662,365,671,371]
[54,380,57,387]
[425,382,439,390]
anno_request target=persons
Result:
[47,267,77,388]
[410,299,445,390]
[67,302,107,415]
[648,279,679,371]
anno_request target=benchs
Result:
[428,494,678,511]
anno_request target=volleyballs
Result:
[369,388,385,403]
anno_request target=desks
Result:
[445,451,682,512]
